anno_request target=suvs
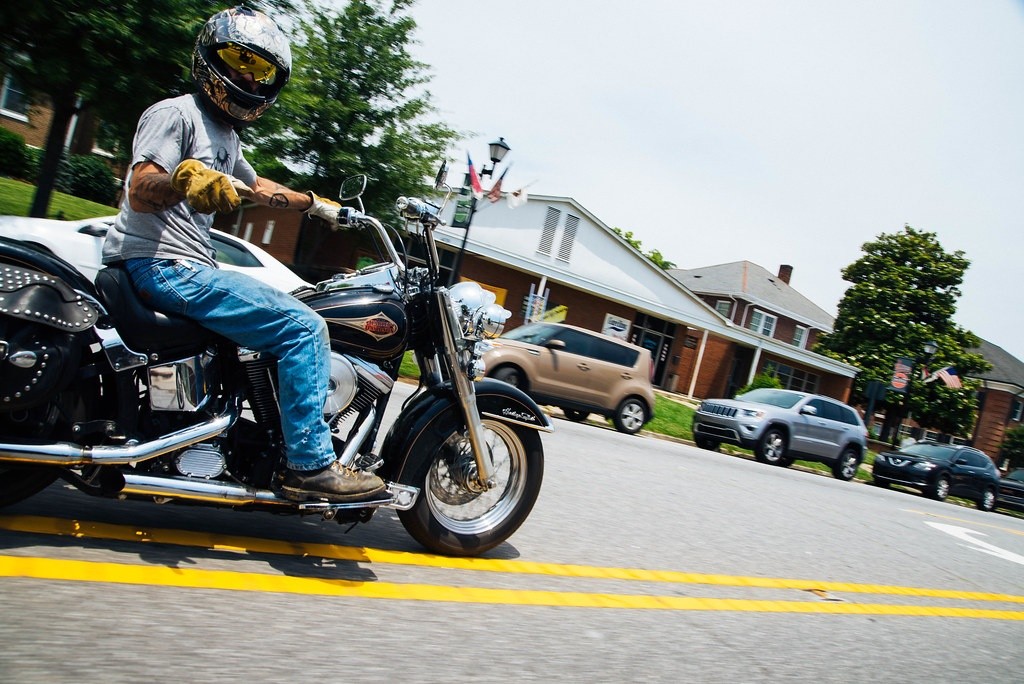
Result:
[692,387,869,482]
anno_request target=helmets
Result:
[192,5,293,127]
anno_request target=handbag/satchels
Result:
[0,263,99,408]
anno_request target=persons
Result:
[102,6,386,502]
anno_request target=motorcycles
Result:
[0,172,552,560]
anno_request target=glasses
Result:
[214,36,288,86]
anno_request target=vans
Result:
[471,320,657,436]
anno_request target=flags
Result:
[468,155,528,210]
[936,366,963,389]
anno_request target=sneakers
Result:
[281,460,387,502]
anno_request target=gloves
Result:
[298,190,354,231]
[170,159,255,215]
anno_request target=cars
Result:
[998,466,1024,513]
[872,437,1000,512]
[0,208,317,318]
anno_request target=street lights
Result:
[446,135,511,287]
